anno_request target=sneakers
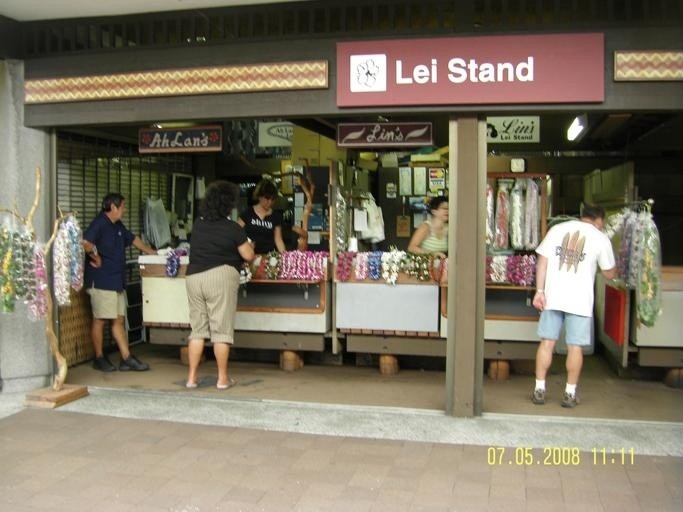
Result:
[119,354,149,371]
[92,357,116,372]
[529,384,545,404]
[560,392,580,408]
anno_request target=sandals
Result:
[185,377,202,388]
[216,375,238,389]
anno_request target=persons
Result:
[185,181,256,388]
[237,179,287,255]
[531,205,620,407]
[408,195,448,259]
[81,192,158,371]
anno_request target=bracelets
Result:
[536,289,545,295]
